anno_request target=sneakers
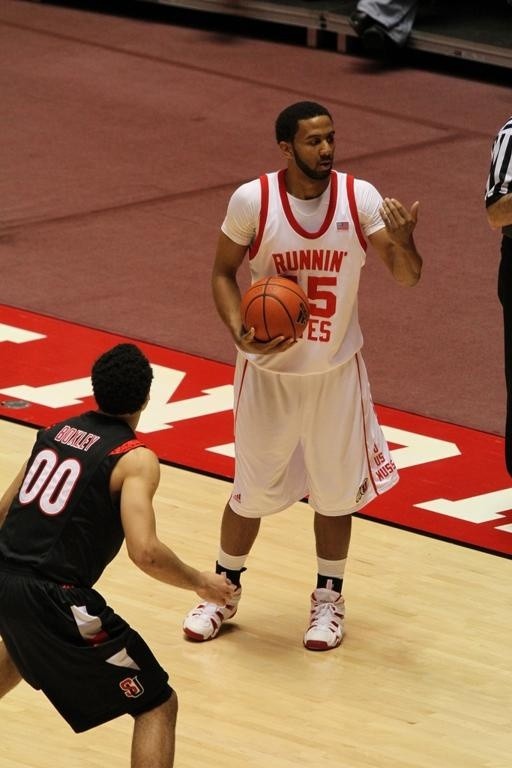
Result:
[181,577,242,643]
[303,588,346,651]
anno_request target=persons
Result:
[0,344,234,768]
[484,115,512,477]
[182,101,422,651]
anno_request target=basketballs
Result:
[239,275,310,342]
